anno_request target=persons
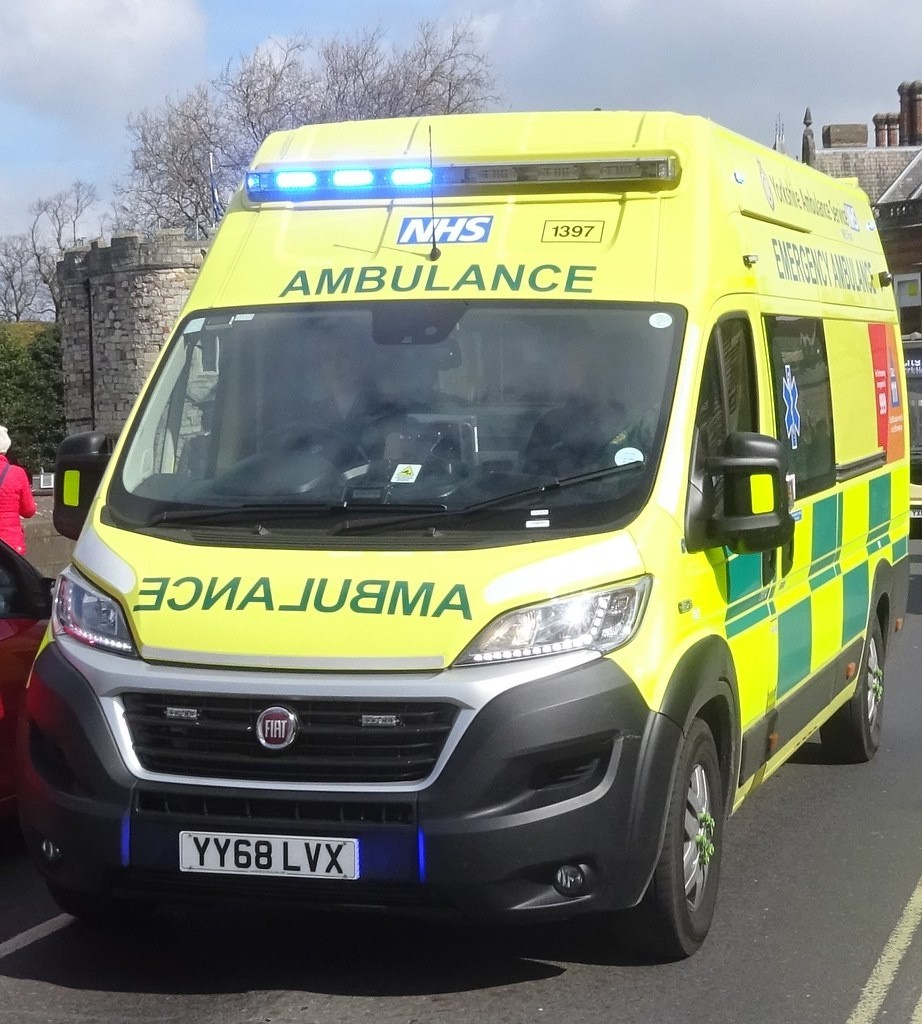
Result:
[0,423,37,559]
[526,341,659,490]
[275,324,403,472]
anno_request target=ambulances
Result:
[18,111,909,959]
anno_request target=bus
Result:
[901,332,921,519]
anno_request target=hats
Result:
[0,425,12,451]
